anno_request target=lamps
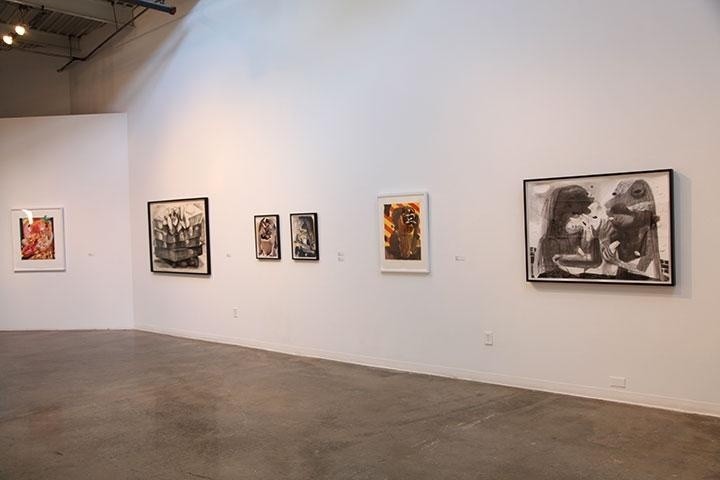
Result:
[2,23,26,45]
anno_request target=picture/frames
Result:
[377,190,431,274]
[289,212,319,260]
[11,206,66,273]
[254,214,281,259]
[147,196,211,275]
[522,168,675,287]
